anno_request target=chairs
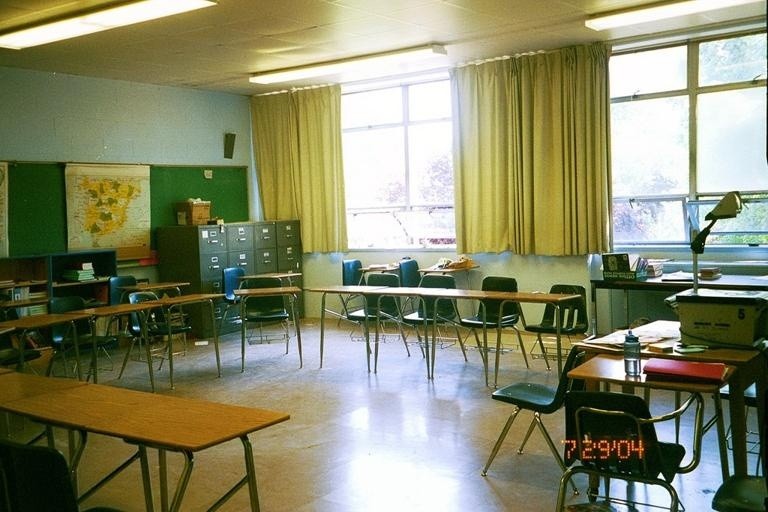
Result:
[305,258,588,385]
[0,276,229,390]
[712,472,767,512]
[483,337,586,494]
[553,386,704,512]
[219,268,247,343]
[0,439,120,512]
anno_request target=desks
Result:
[590,274,767,338]
[564,319,767,489]
[234,273,303,353]
[0,372,290,512]
[232,287,304,372]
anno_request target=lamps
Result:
[689,190,742,291]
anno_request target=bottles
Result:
[623,330,642,376]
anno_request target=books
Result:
[643,357,728,382]
[581,316,684,350]
[0,261,98,348]
[596,251,673,281]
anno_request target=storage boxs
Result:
[675,291,762,348]
[175,199,211,226]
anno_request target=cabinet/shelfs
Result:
[156,219,305,339]
[0,247,117,353]
